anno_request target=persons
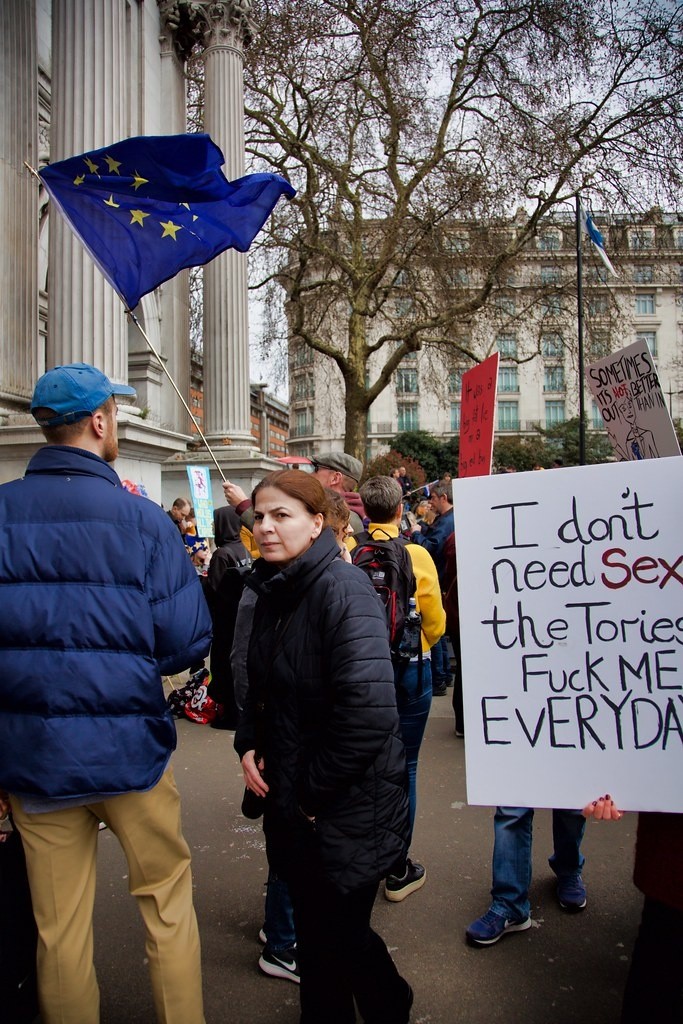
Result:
[168,447,683,1024]
[0,359,212,1024]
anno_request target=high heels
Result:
[385,858,426,901]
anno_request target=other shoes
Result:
[432,676,454,696]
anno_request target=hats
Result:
[30,362,136,427]
[313,452,363,483]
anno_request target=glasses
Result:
[312,463,337,472]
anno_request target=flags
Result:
[38,130,296,311]
[581,201,621,279]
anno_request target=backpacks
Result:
[349,530,423,696]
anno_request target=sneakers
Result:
[466,910,532,944]
[258,921,300,983]
[557,875,587,908]
